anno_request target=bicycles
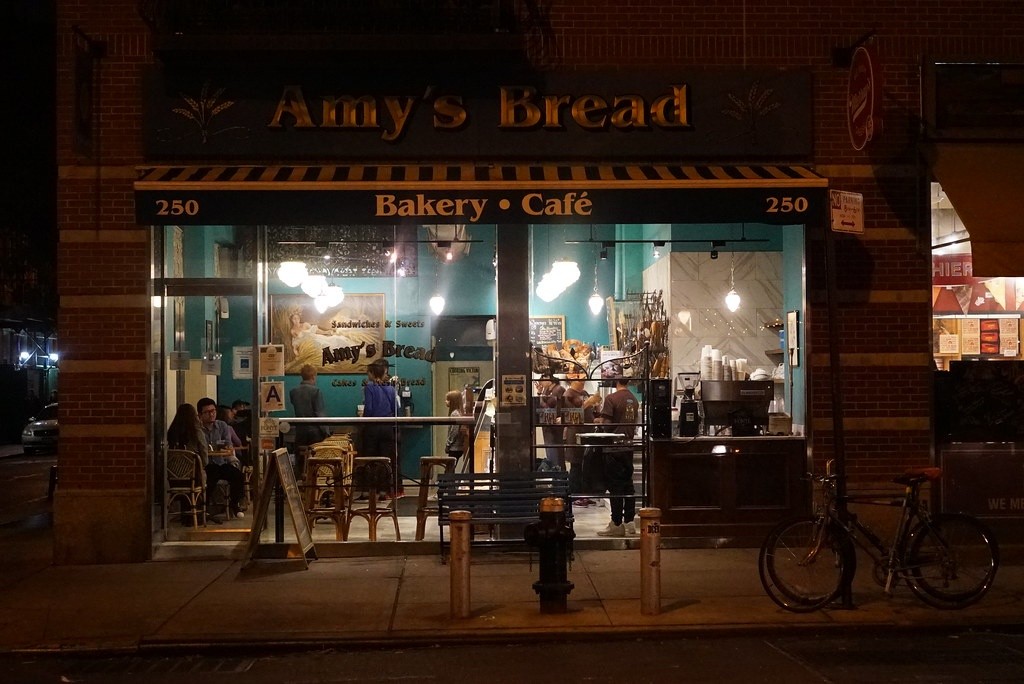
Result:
[759,458,1002,613]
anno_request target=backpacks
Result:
[457,425,469,452]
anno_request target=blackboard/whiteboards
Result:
[244,448,315,554]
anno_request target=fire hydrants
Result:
[523,496,577,614]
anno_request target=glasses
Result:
[201,409,216,415]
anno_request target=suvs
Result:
[21,403,59,455]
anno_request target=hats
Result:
[231,399,251,409]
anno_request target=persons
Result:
[289,365,330,481]
[49,389,58,403]
[158,398,252,518]
[594,378,639,536]
[427,389,469,501]
[535,374,567,471]
[563,370,606,507]
[357,358,405,501]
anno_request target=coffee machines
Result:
[678,372,700,438]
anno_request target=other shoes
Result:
[233,506,245,518]
[573,498,597,507]
[427,492,442,501]
[353,493,370,503]
[385,489,405,499]
[378,495,386,502]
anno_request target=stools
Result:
[344,456,401,543]
[305,458,346,542]
[413,455,457,541]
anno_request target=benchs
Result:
[437,471,574,565]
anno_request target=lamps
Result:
[536,224,580,302]
[429,225,445,316]
[725,224,741,313]
[652,245,661,258]
[599,250,608,260]
[588,223,604,315]
[932,183,961,256]
[711,250,720,260]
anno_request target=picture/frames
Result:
[267,293,383,375]
[786,309,801,368]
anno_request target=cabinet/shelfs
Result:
[528,339,649,507]
[764,319,784,384]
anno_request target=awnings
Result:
[922,142,1024,277]
[133,164,828,226]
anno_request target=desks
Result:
[207,449,231,456]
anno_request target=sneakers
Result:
[596,520,625,537]
[623,521,636,534]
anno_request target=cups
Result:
[701,345,748,382]
[357,405,365,416]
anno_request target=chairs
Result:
[168,432,354,528]
